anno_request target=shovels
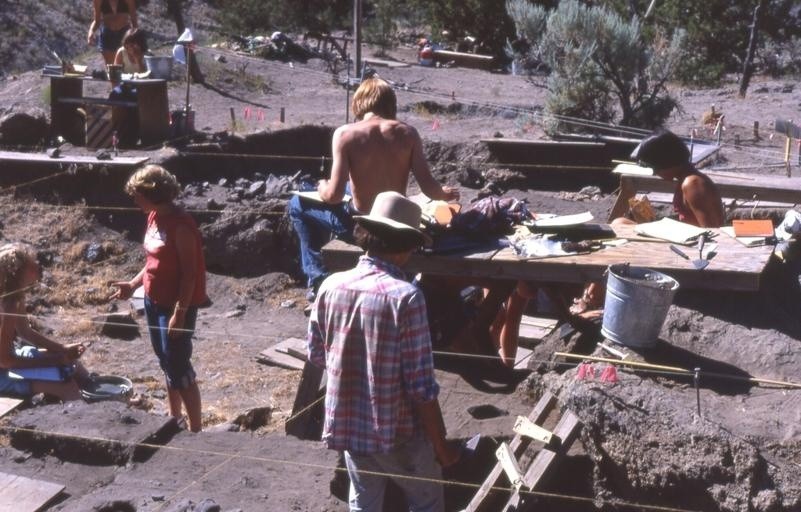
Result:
[692,234,709,272]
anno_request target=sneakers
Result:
[306,286,318,302]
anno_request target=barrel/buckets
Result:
[79,374,133,407]
[600,263,681,349]
[144,56,174,80]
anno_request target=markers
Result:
[670,245,689,260]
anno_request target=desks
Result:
[309,195,787,395]
[41,69,172,146]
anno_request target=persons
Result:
[0,243,88,402]
[568,131,724,316]
[108,27,151,101]
[88,1,138,71]
[106,163,210,433]
[480,276,541,371]
[305,190,460,512]
[290,77,460,317]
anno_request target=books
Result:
[511,210,775,260]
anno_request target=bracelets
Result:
[174,301,188,313]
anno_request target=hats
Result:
[353,191,435,247]
